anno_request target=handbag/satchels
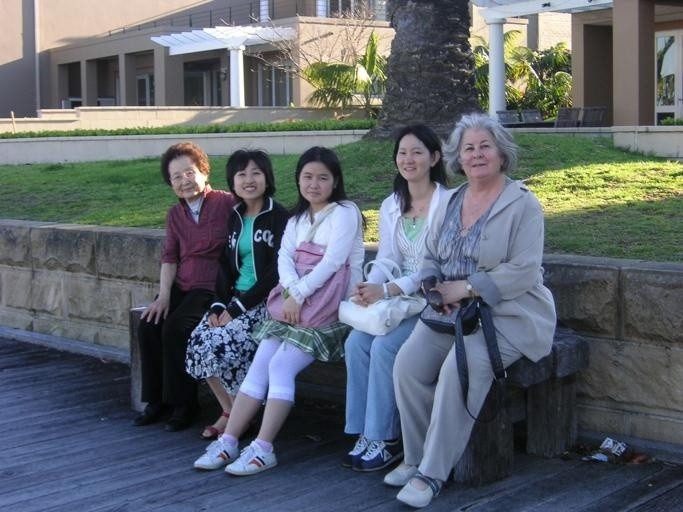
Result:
[419,299,507,423]
[267,240,351,328]
[337,258,426,336]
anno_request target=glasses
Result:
[169,169,195,182]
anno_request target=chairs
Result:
[520,108,545,126]
[496,109,524,127]
[553,107,581,127]
[579,106,609,127]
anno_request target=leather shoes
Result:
[130,404,167,426]
[161,410,185,431]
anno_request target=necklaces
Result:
[191,196,204,216]
[407,184,436,230]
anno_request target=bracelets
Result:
[383,282,390,300]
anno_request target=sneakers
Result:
[224,441,278,476]
[383,461,418,486]
[193,437,239,468]
[341,435,371,467]
[395,473,443,508]
[352,440,404,472]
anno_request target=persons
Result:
[198,147,363,481]
[335,121,451,475]
[133,142,237,430]
[185,149,292,441]
[384,110,557,509]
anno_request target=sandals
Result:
[199,412,230,440]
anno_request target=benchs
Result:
[127,304,590,483]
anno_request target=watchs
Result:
[466,280,475,300]
